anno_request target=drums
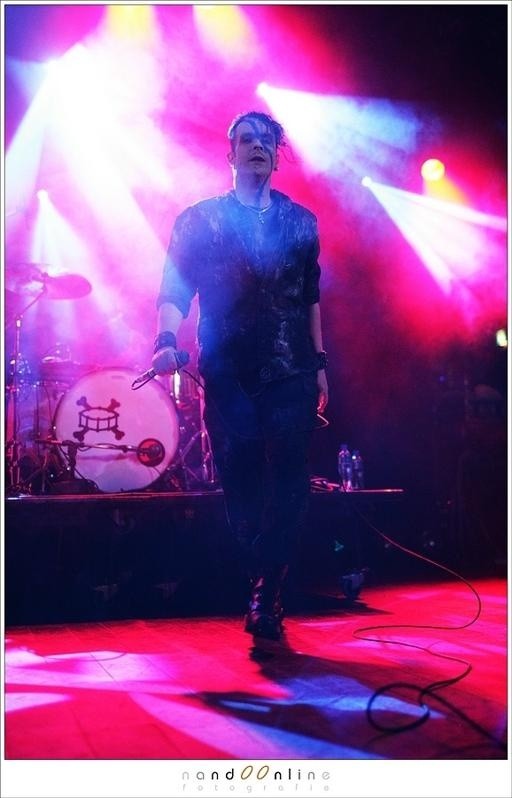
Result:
[51,367,186,494]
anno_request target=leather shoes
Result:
[244,593,285,640]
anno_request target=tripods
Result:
[5,317,65,495]
[153,376,219,492]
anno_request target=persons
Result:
[150,106,333,661]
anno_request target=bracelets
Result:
[314,351,329,371]
[151,331,178,353]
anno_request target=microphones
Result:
[135,351,188,383]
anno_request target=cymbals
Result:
[4,262,92,300]
